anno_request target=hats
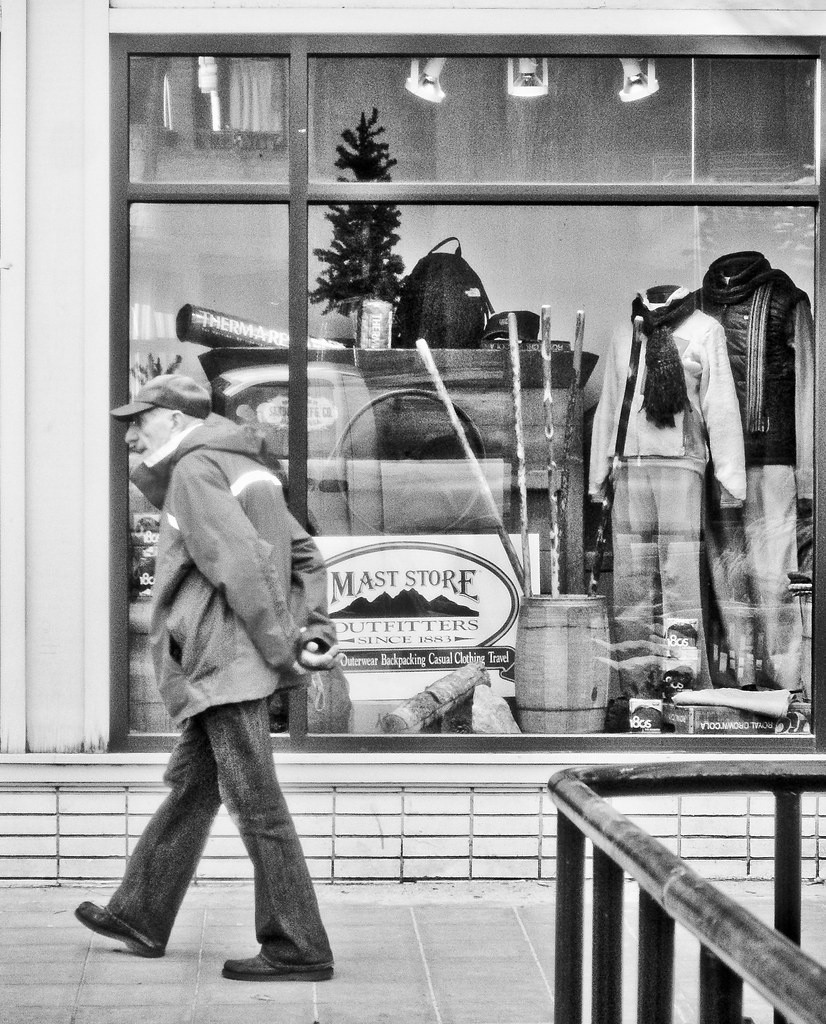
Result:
[109,374,212,419]
[483,310,541,342]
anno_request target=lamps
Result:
[618,58,660,103]
[405,57,449,103]
[507,58,549,98]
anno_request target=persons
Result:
[74,373,341,981]
[589,251,815,723]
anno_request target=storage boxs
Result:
[663,702,811,735]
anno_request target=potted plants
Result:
[308,107,402,353]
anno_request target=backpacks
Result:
[394,237,494,348]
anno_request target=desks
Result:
[198,351,598,593]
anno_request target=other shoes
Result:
[76,901,166,959]
[222,954,334,981]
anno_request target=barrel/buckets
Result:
[516,593,612,732]
